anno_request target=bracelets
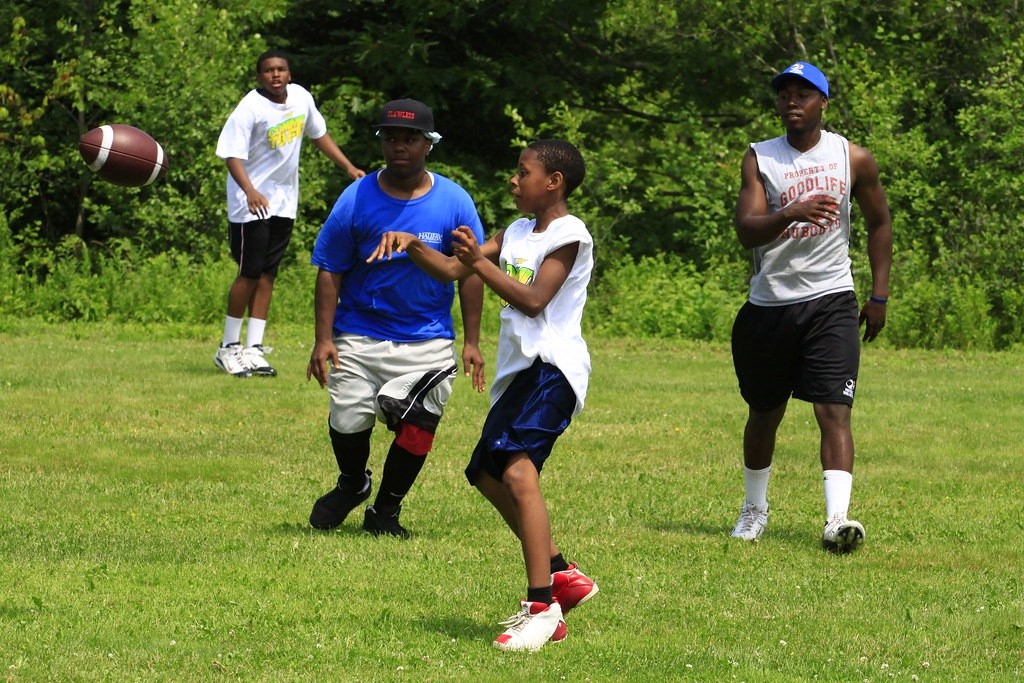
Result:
[870,296,888,303]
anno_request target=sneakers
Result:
[730,501,769,541]
[243,344,278,377]
[822,513,865,555]
[308,469,371,530]
[494,596,567,652]
[363,505,410,541]
[213,341,253,378]
[549,561,599,616]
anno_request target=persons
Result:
[217,49,367,380]
[306,96,486,541]
[729,63,894,558]
[366,140,601,653]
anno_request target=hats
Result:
[372,97,436,131]
[770,62,829,98]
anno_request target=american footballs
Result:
[79,125,169,186]
